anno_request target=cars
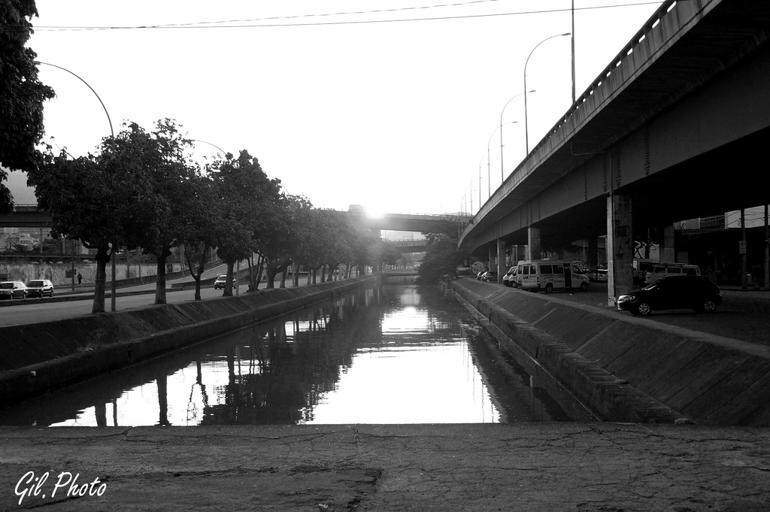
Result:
[613,272,724,319]
[0,280,29,300]
[24,279,55,297]
[213,273,236,290]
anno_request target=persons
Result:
[77,272,83,284]
[476,271,483,279]
[481,271,490,282]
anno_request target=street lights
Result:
[523,31,573,157]
[456,88,537,234]
[27,58,118,152]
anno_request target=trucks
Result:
[475,253,705,295]
